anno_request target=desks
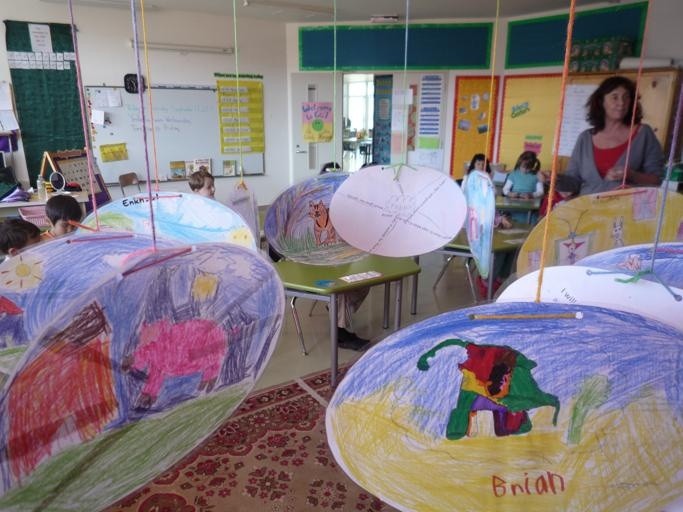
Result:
[0,190,89,221]
[343,136,373,159]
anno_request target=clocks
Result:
[123,74,146,94]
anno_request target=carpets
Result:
[106,361,405,512]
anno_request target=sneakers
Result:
[336,327,370,350]
[493,279,502,294]
[476,276,488,302]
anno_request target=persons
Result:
[189,172,215,199]
[269,244,373,350]
[40,196,83,239]
[461,154,492,193]
[321,162,340,174]
[559,77,666,202]
[502,150,545,226]
[0,219,40,263]
[475,208,517,299]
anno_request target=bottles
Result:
[36,174,48,202]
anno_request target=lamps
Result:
[131,39,234,55]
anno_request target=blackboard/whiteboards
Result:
[84,85,265,186]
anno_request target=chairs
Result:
[120,173,142,196]
